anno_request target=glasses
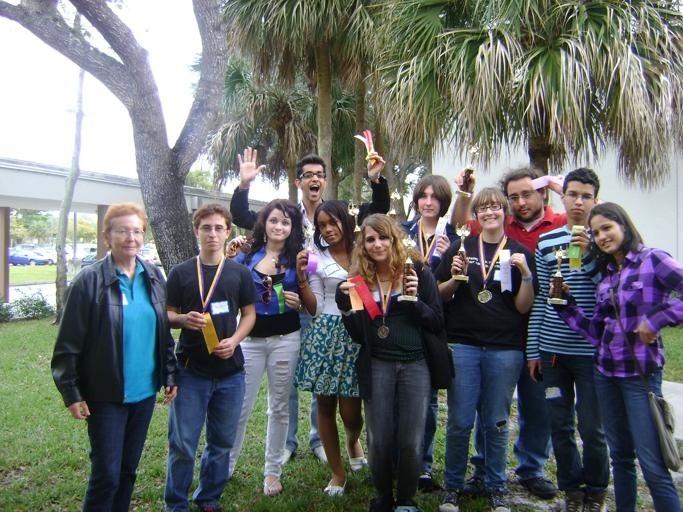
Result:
[259,276,273,305]
[473,203,502,214]
[299,171,324,180]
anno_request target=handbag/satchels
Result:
[650,389,683,473]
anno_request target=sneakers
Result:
[312,445,329,463]
[439,487,460,511]
[487,491,511,512]
[368,495,418,512]
[418,471,432,492]
[282,449,293,465]
[464,478,485,494]
[561,488,608,512]
[200,503,222,511]
[517,476,556,499]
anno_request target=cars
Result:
[8,245,45,266]
[81,255,97,268]
[33,249,58,265]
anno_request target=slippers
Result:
[263,476,283,497]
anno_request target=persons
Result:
[50,202,179,512]
[228,146,610,512]
[548,202,683,511]
[165,201,262,512]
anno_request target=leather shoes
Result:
[345,439,367,472]
[324,471,348,496]
